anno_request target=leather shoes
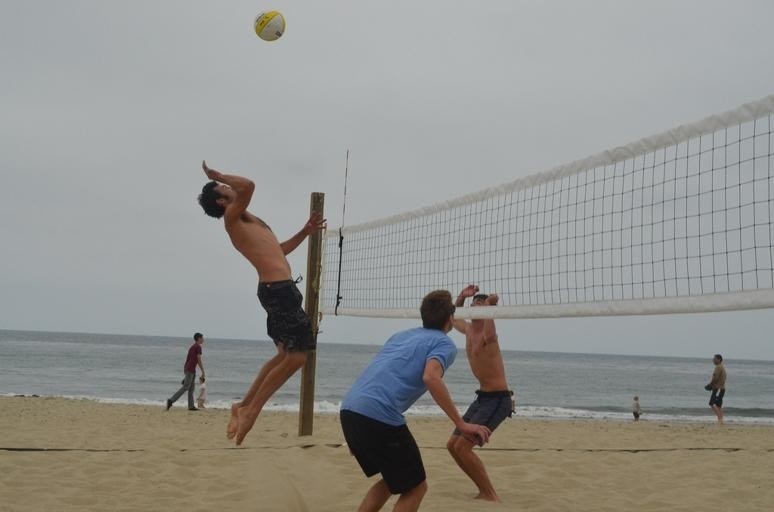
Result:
[188,407,199,410]
[167,398,172,410]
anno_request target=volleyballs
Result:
[255,9,285,41]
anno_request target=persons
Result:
[339,289,492,512]
[165,333,205,412]
[632,396,643,422]
[198,161,327,447]
[196,377,206,409]
[446,285,513,503]
[704,354,726,426]
